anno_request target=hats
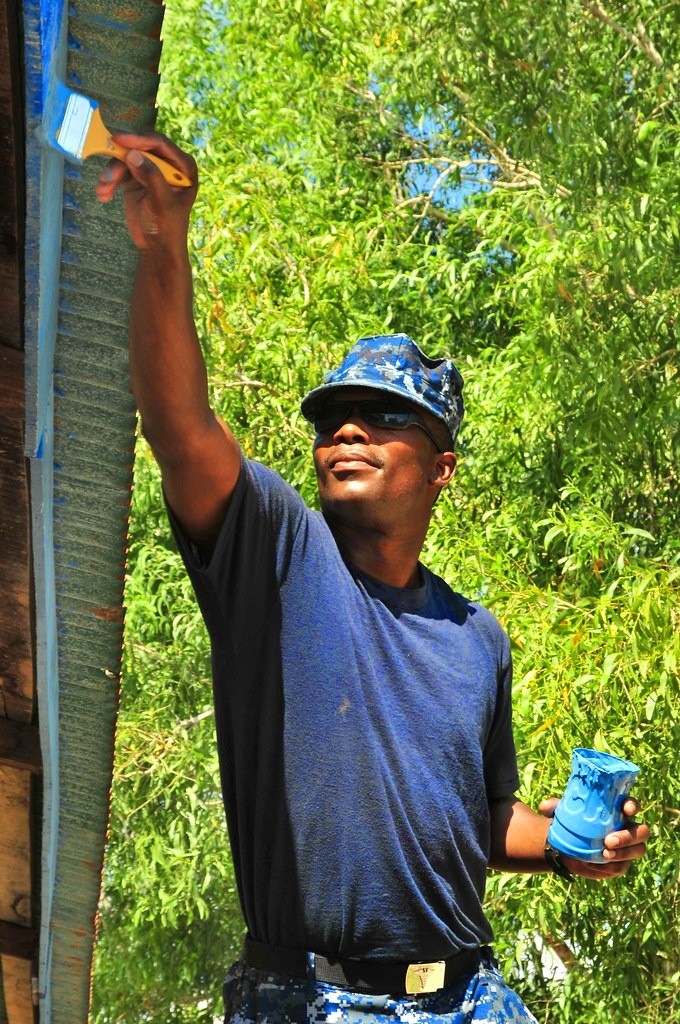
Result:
[301,333,465,445]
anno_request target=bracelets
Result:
[543,836,578,883]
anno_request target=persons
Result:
[98,135,649,1024]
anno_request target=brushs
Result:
[39,77,193,199]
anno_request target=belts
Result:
[240,939,479,994]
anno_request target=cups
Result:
[547,747,642,865]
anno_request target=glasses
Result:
[314,400,443,453]
